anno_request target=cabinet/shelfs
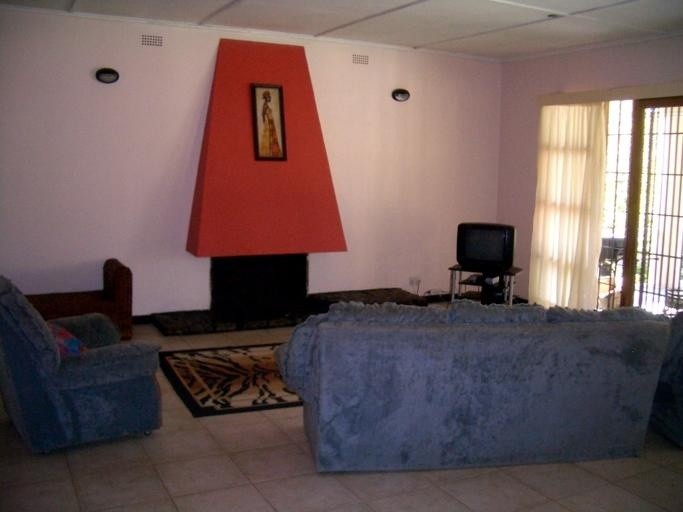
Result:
[447,264,523,306]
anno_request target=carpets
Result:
[159,342,303,420]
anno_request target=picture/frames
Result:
[250,83,288,161]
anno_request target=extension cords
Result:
[430,288,449,295]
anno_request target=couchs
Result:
[273,299,672,476]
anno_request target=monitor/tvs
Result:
[457,223,516,266]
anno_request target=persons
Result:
[256,89,282,158]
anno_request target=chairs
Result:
[0,273,162,456]
